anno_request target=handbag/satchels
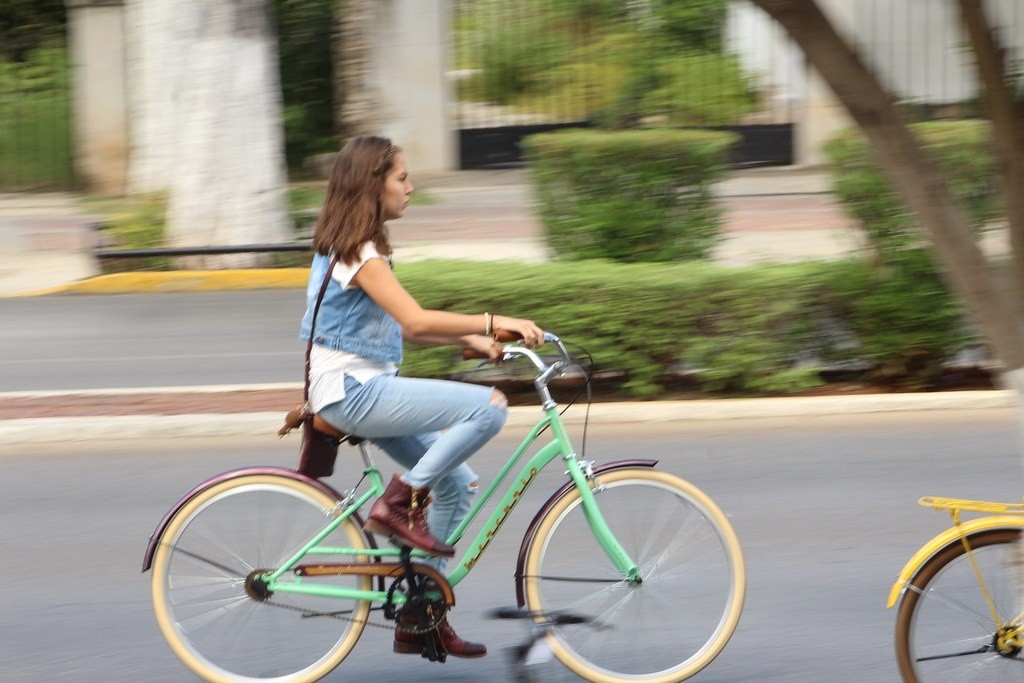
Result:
[291,431,340,480]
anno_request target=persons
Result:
[297,136,545,660]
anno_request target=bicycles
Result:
[882,494,1023,683]
[138,323,748,682]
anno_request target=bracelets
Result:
[491,314,493,333]
[484,312,489,336]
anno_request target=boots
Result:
[362,473,460,561]
[389,598,490,661]
